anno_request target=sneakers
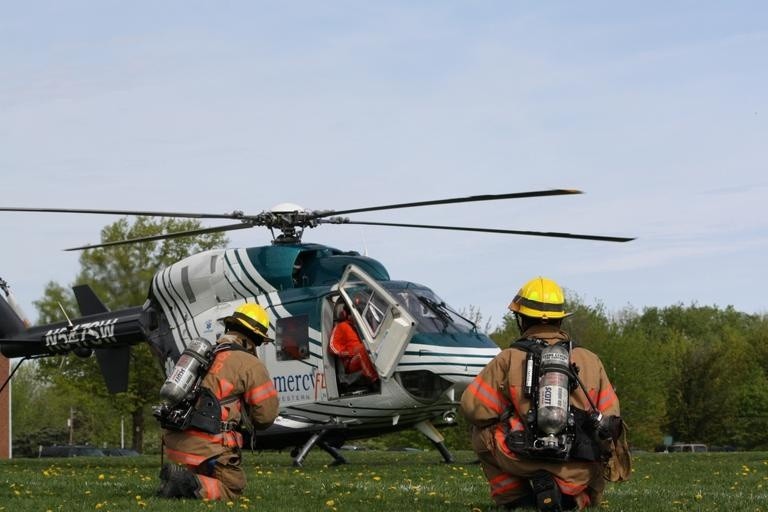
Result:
[370,378,381,391]
[155,464,197,498]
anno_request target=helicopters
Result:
[0,187,638,467]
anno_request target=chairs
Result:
[335,354,366,392]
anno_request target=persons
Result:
[160,304,278,499]
[462,279,631,511]
[330,303,381,393]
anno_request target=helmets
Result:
[225,303,276,342]
[508,277,566,319]
[334,302,346,320]
